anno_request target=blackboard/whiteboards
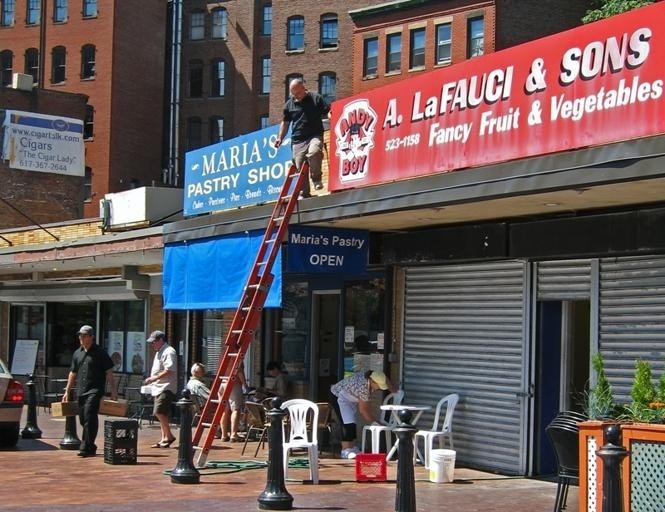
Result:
[11,339,40,376]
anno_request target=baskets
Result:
[356,452,386,481]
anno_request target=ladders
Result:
[191,160,311,469]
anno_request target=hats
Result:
[370,371,389,390]
[77,325,93,336]
[146,331,164,343]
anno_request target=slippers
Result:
[152,437,175,448]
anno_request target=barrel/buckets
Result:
[429,447,456,485]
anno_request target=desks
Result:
[412,392,462,469]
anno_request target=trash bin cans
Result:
[104,418,139,465]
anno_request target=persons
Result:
[217,344,251,442]
[257,359,288,397]
[186,362,222,438]
[61,325,118,457]
[273,80,331,198]
[143,329,180,449]
[329,369,398,459]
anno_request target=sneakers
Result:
[214,432,245,442]
[314,178,323,189]
[78,450,96,456]
[341,447,360,459]
[303,193,311,198]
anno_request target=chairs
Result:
[122,380,252,437]
[276,398,321,486]
[357,387,404,458]
[237,390,335,458]
[544,407,590,512]
[377,402,433,467]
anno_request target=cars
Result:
[0,359,24,448]
